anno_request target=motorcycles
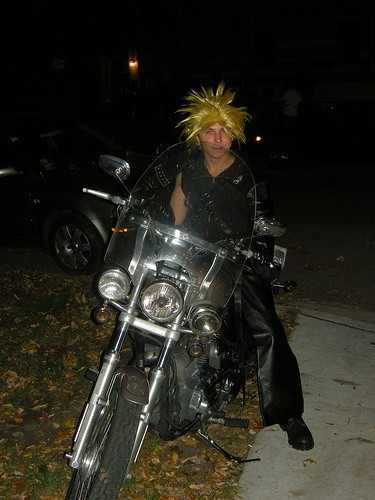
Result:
[66,154,289,500]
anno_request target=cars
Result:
[1,111,165,277]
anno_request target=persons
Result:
[128,80,314,452]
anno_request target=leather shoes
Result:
[280,416,315,450]
